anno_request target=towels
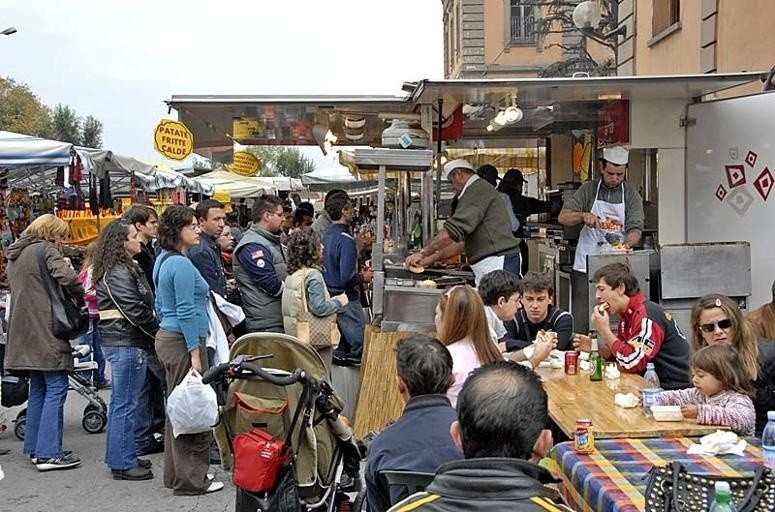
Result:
[687,431,747,457]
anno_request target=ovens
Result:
[523,188,583,240]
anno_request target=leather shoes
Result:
[112,437,164,480]
[206,443,225,493]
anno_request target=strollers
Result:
[202,332,362,512]
[4,344,107,441]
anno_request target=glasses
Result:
[269,211,285,218]
[698,319,731,332]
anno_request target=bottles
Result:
[762,409,775,475]
[587,334,603,383]
[641,362,661,421]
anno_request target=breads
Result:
[536,329,549,341]
[409,265,424,272]
[598,302,610,312]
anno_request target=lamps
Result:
[379,102,441,147]
[573,2,627,39]
[488,93,525,134]
[312,109,368,154]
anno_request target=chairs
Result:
[376,470,440,508]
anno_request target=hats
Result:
[443,159,478,178]
[603,145,629,165]
[479,165,528,183]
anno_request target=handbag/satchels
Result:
[295,268,339,349]
[641,462,775,512]
[231,428,302,512]
[52,284,89,339]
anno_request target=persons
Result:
[365,335,463,512]
[384,362,580,511]
[451,165,497,221]
[0,186,398,496]
[640,343,757,438]
[499,167,554,281]
[403,157,525,285]
[689,294,774,442]
[507,269,576,351]
[558,146,647,337]
[571,263,695,392]
[431,284,503,410]
[477,269,559,372]
[746,281,775,343]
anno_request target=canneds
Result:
[574,420,593,454]
[565,351,578,376]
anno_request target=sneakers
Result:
[31,451,81,471]
